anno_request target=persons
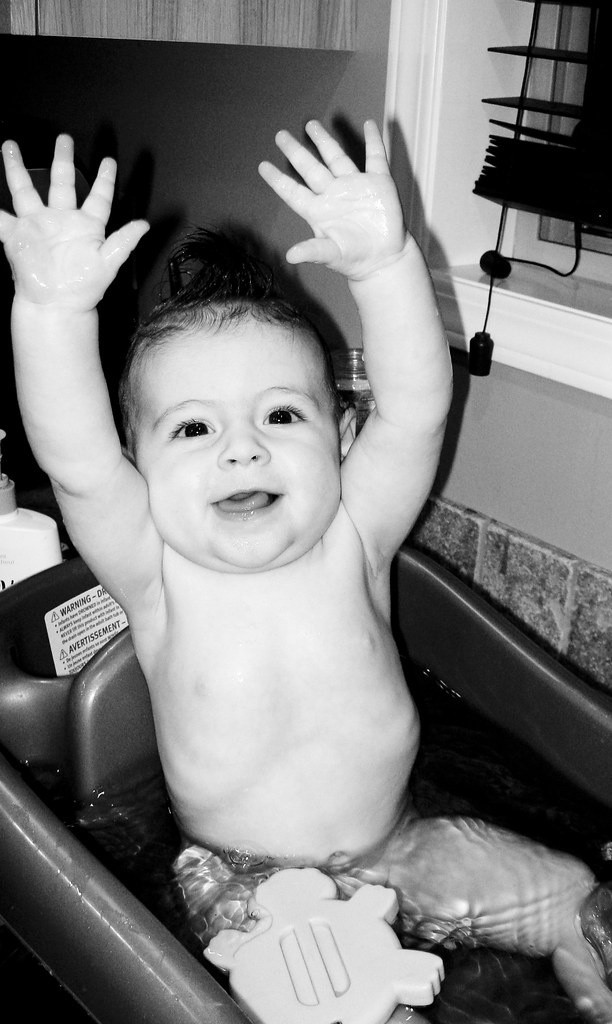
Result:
[0,117,612,1024]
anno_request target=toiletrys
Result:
[0,429,65,596]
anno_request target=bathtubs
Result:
[0,529,612,1024]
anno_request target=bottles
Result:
[0,472,62,589]
